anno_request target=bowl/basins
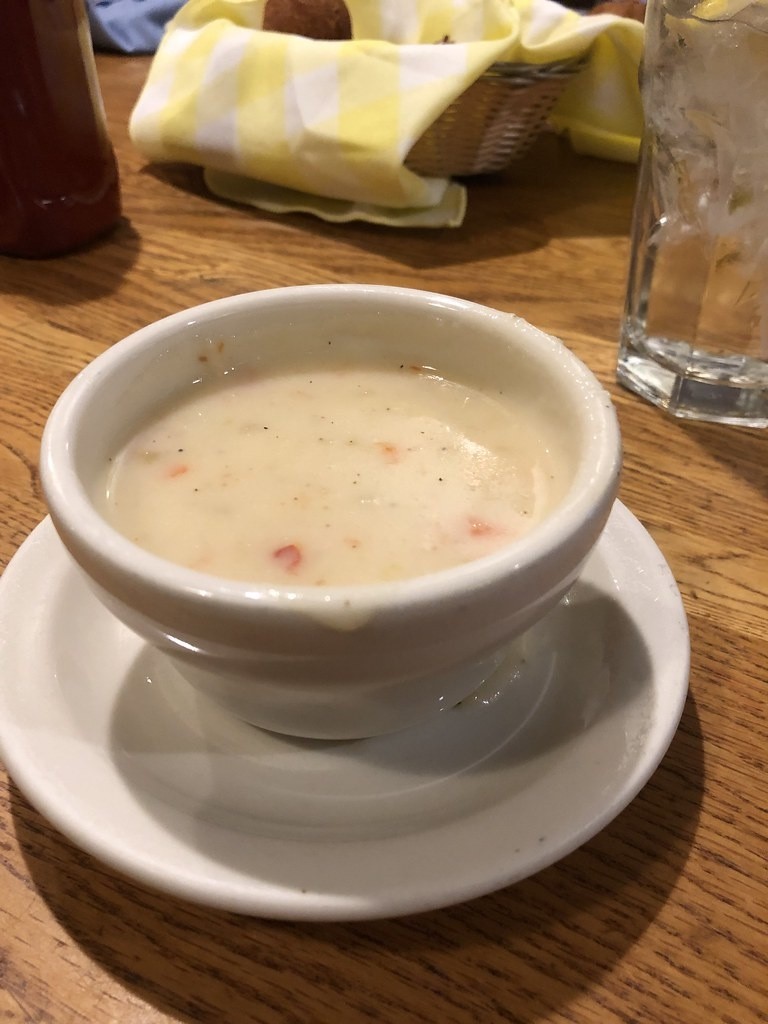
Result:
[43,284,619,740]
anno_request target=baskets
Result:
[403,54,591,177]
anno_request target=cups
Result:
[614,0,768,427]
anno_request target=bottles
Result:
[0,0,121,260]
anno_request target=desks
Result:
[0,49,768,1024]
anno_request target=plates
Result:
[0,498,691,923]
